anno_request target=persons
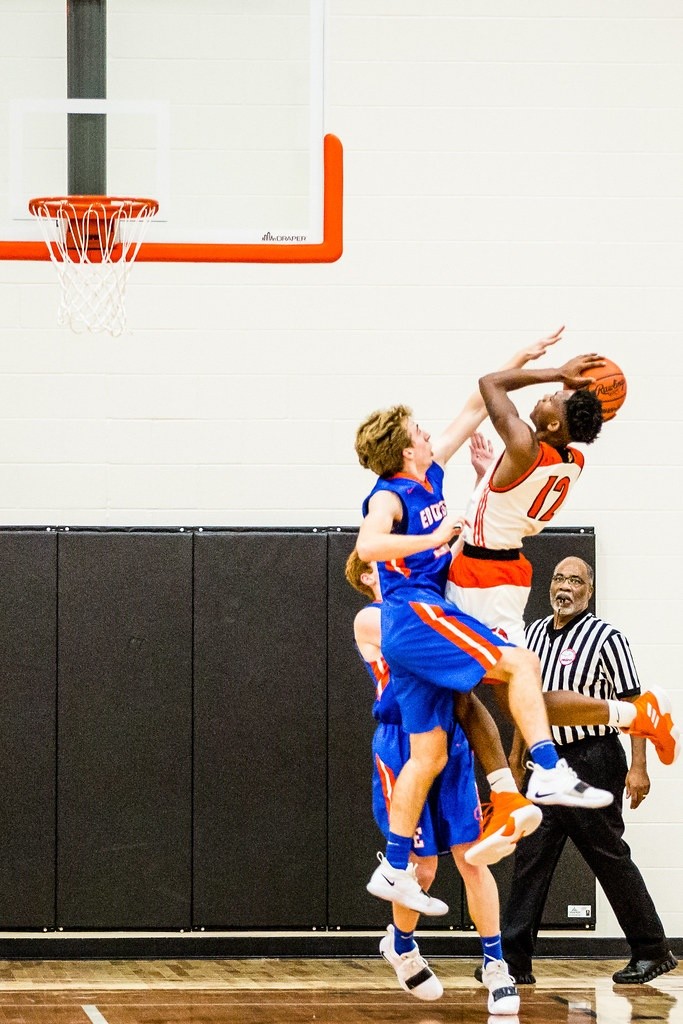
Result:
[345,550,521,1017]
[461,353,676,868]
[474,554,680,985]
[358,325,613,917]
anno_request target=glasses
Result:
[551,575,592,588]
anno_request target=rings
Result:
[642,795,647,798]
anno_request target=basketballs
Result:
[562,357,627,422]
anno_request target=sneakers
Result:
[474,959,536,985]
[611,948,678,984]
[379,924,444,1002]
[482,961,520,1015]
[463,790,543,866]
[524,758,615,810]
[619,690,676,765]
[365,850,450,917]
